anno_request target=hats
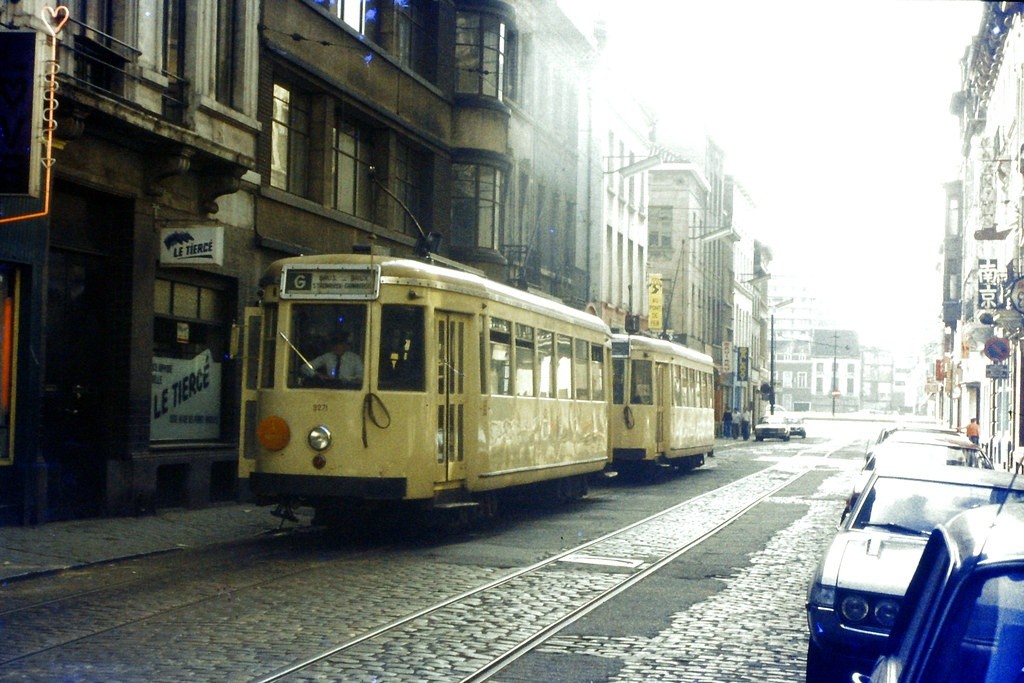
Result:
[329,329,349,342]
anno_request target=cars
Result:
[805,412,1024,683]
[765,404,789,417]
[787,414,806,439]
[849,498,1024,683]
[755,415,791,442]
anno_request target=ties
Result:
[333,355,342,383]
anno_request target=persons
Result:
[732,407,741,440]
[966,418,980,455]
[721,405,733,440]
[740,406,751,441]
[300,334,363,385]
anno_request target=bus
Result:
[228,250,613,538]
[611,331,717,481]
